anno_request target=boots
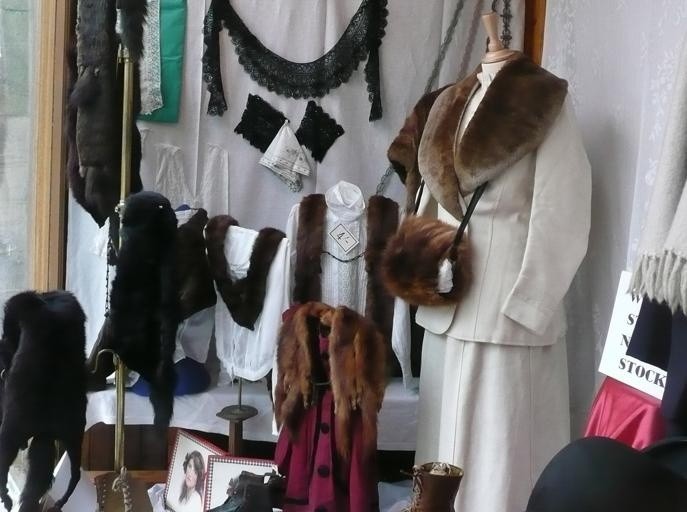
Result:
[121,475,154,512]
[94,470,126,512]
[397,460,465,512]
[205,468,288,511]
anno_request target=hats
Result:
[522,432,687,512]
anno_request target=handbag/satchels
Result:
[377,213,478,309]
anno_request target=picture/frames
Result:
[163,428,229,512]
[204,455,286,512]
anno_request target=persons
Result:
[179,450,208,509]
[415,15,593,512]
